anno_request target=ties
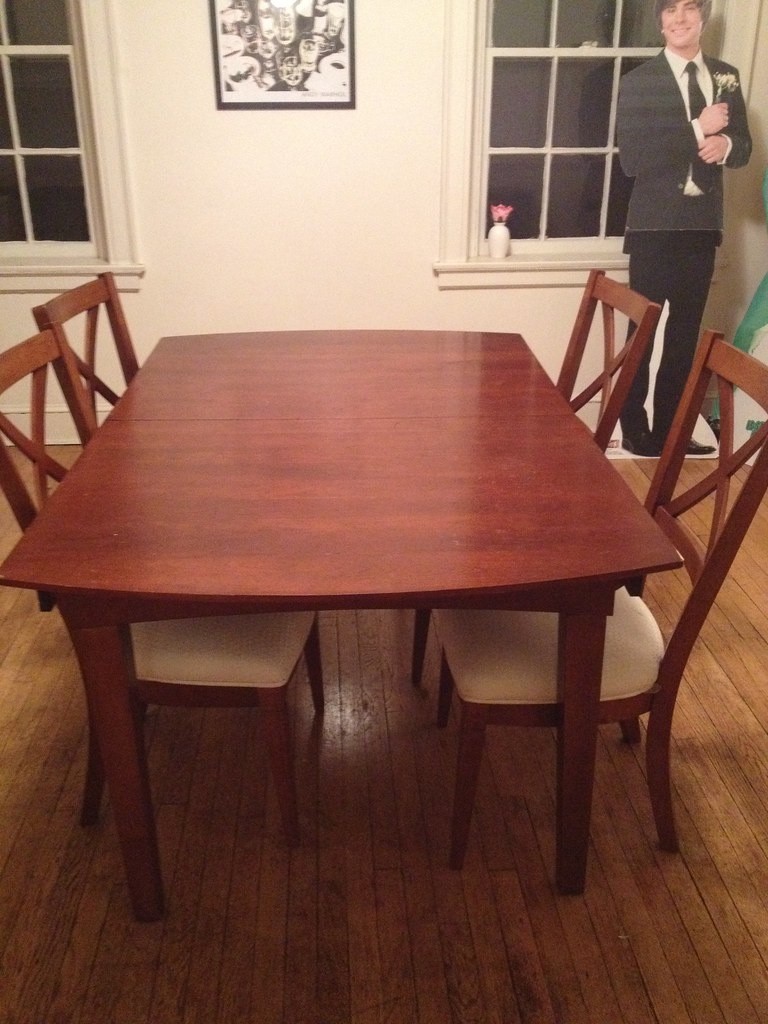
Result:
[683,60,714,195]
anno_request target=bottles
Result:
[488,222,511,261]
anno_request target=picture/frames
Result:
[209,0,355,112]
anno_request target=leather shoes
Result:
[686,439,717,458]
[621,432,662,457]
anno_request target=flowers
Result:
[490,204,513,223]
[713,70,739,100]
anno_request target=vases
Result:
[488,222,510,258]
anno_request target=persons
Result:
[615,0,753,457]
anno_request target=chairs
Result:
[0,329,325,849]
[411,268,661,690]
[32,271,140,427]
[435,327,768,871]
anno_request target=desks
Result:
[0,329,682,920]
